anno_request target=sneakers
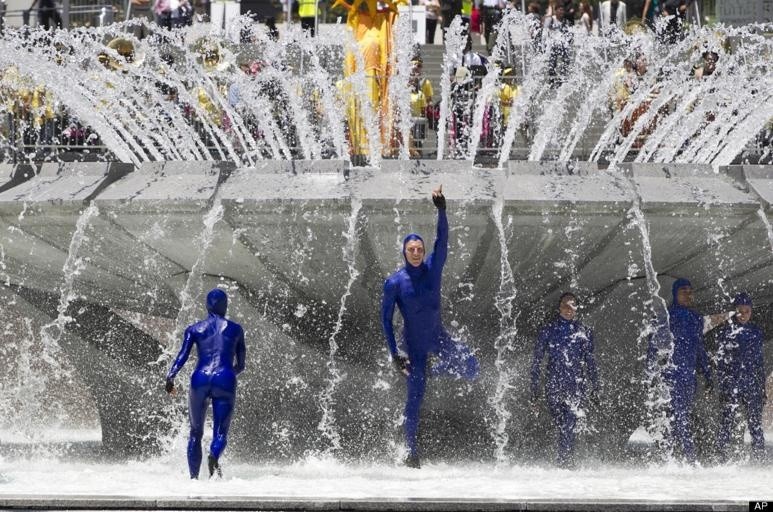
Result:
[404,456,419,469]
[208,455,221,479]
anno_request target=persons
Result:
[717,293,765,464]
[529,292,600,469]
[5,0,721,159]
[165,288,245,478]
[380,183,479,469]
[646,277,713,466]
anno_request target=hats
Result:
[206,289,226,315]
[672,278,691,307]
[733,294,753,323]
[403,234,424,264]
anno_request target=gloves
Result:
[165,379,173,393]
[432,193,444,209]
[391,354,406,370]
[704,380,714,394]
